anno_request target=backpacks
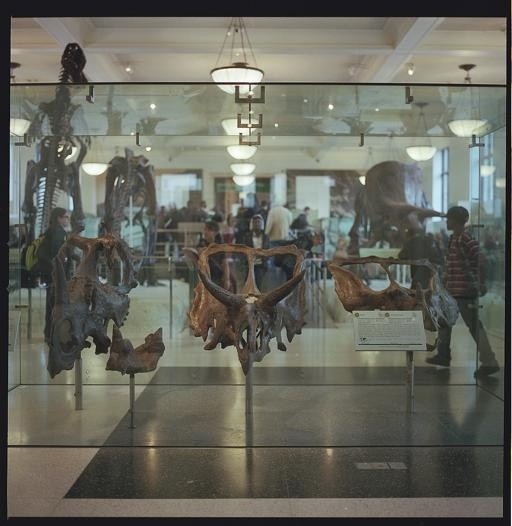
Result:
[20,223,69,290]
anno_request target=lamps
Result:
[404,64,492,164]
[10,63,121,176]
[210,17,264,189]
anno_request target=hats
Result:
[440,206,469,223]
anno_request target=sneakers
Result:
[426,356,451,367]
[474,365,500,375]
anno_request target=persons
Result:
[241,215,271,291]
[398,228,434,289]
[159,196,316,247]
[199,221,225,288]
[38,206,80,342]
[426,206,501,377]
